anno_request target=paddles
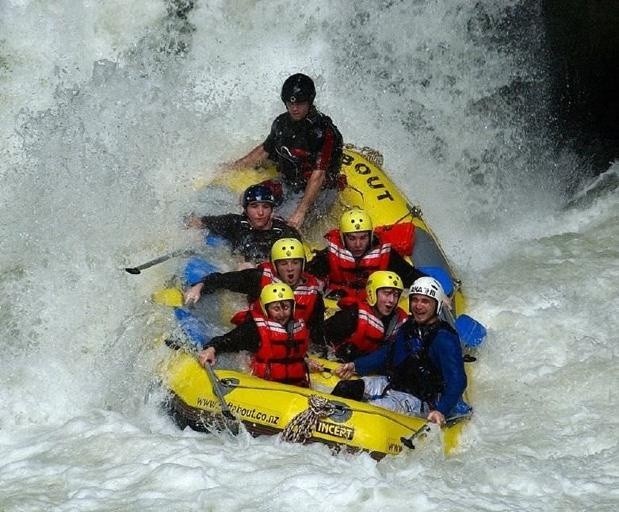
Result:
[124,232,230,276]
[174,308,236,420]
[454,314,486,355]
[417,264,476,362]
[165,257,225,350]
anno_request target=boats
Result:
[146,146,478,463]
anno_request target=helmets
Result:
[340,210,373,247]
[259,283,295,319]
[272,238,305,276]
[243,184,275,207]
[409,277,444,315]
[366,271,404,311]
[281,73,316,104]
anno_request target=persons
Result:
[182,239,336,361]
[313,209,419,308]
[324,271,411,363]
[199,283,324,389]
[336,277,467,426]
[212,73,343,230]
[187,185,303,262]
